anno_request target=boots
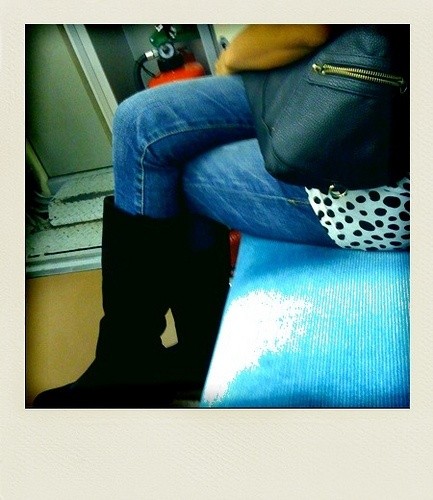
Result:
[32,197,166,409]
[155,208,230,400]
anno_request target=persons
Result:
[32,24,410,409]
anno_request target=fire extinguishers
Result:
[132,24,242,266]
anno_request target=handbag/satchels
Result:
[235,25,410,190]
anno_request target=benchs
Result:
[199,231,410,408]
